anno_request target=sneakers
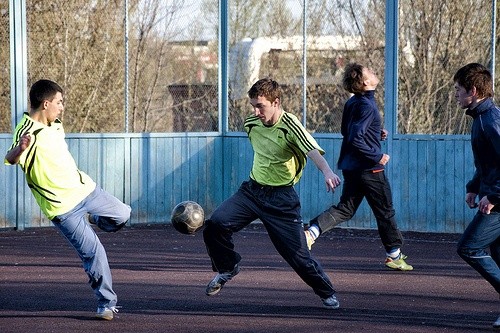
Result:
[206,264,240,296]
[95,305,122,321]
[384,252,413,271]
[322,294,340,309]
[304,230,313,251]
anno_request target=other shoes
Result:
[494,317,500,327]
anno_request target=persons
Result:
[451,63,500,328]
[202,78,342,309]
[303,64,414,271]
[4,79,132,320]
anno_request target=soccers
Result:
[171,201,205,235]
